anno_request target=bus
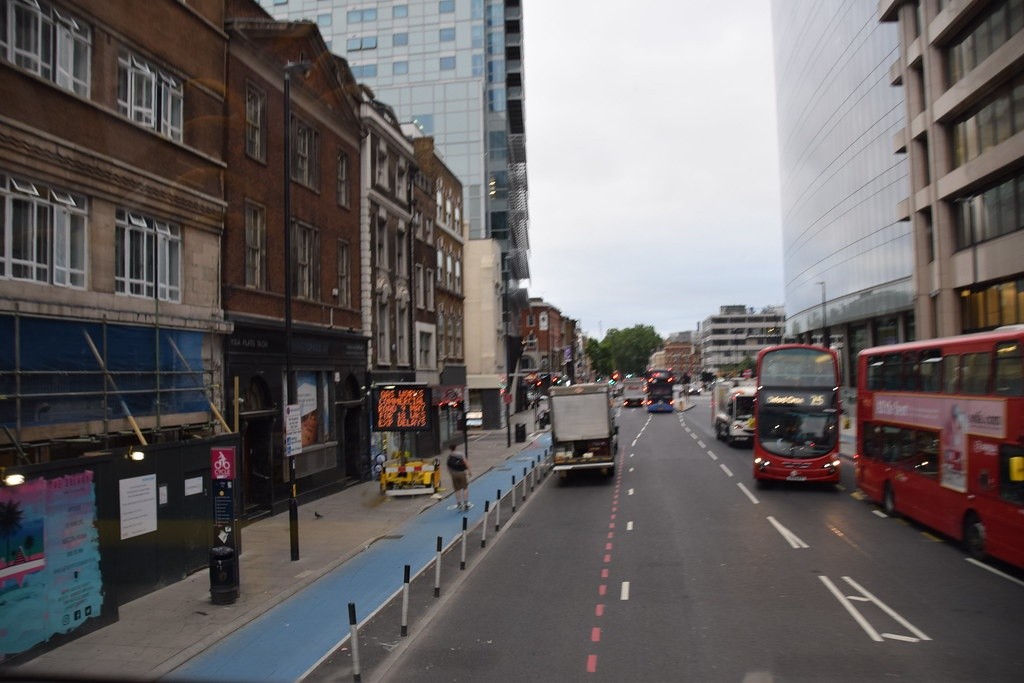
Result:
[847,324,1024,569]
[747,344,850,487]
[646,368,675,412]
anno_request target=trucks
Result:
[548,383,619,480]
[711,378,755,444]
[623,377,645,407]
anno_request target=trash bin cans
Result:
[515,423,526,443]
[209,546,238,604]
[544,409,551,424]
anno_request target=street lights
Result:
[282,61,315,563]
[503,256,513,447]
[815,281,828,347]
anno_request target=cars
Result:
[672,384,701,395]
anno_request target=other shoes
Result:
[457,505,462,513]
[465,504,473,510]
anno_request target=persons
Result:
[447,444,473,511]
[947,365,964,393]
